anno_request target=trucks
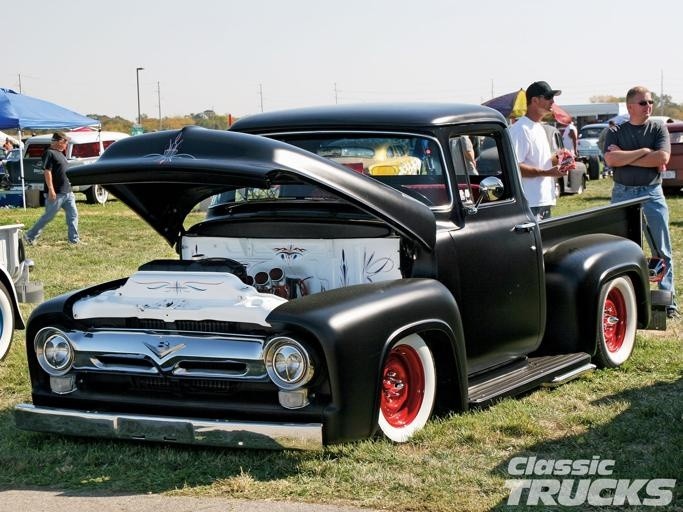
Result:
[0,223,45,362]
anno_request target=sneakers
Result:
[67,240,89,247]
[23,234,39,248]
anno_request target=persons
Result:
[602,86,679,320]
[411,131,497,176]
[562,114,579,156]
[24,129,87,247]
[2,138,12,150]
[595,119,617,179]
[508,80,569,224]
[9,140,19,148]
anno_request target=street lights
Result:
[135,65,144,124]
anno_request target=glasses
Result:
[538,95,555,100]
[628,100,653,107]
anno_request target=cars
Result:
[1,131,133,207]
[16,102,650,457]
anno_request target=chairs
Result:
[399,183,479,204]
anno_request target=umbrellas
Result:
[481,86,571,126]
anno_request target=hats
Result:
[527,81,561,97]
[51,130,71,142]
